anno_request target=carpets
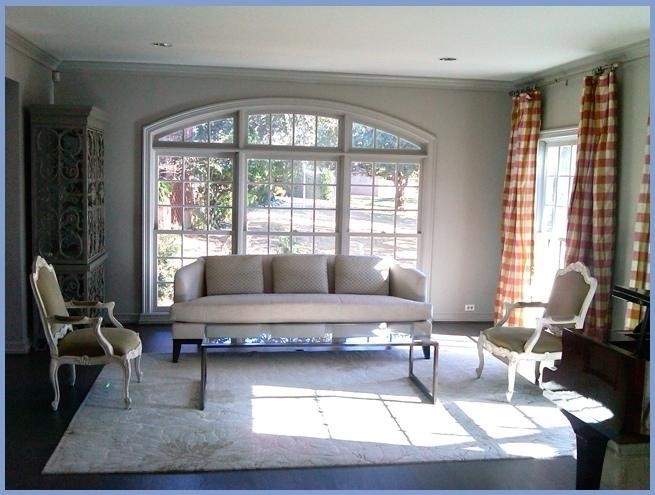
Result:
[41,349,577,474]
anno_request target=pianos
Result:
[541,284,650,490]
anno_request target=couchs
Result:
[169,255,432,362]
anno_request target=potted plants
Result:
[158,179,172,230]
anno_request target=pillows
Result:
[206,255,264,295]
[334,255,390,295]
[272,255,329,293]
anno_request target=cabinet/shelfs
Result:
[30,104,111,352]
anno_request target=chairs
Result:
[476,261,598,402]
[30,255,143,411]
